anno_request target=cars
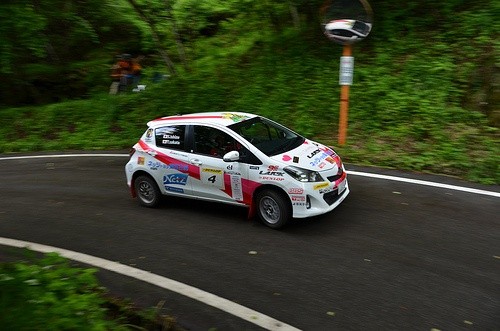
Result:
[125,111,351,229]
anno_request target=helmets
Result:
[207,133,226,150]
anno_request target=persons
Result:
[235,131,257,149]
[211,137,234,157]
